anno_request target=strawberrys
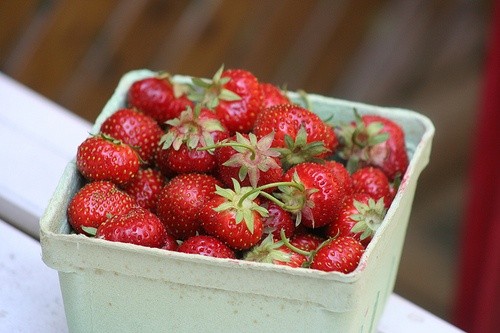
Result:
[67,63,409,274]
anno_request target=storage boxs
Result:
[40,68,435,333]
